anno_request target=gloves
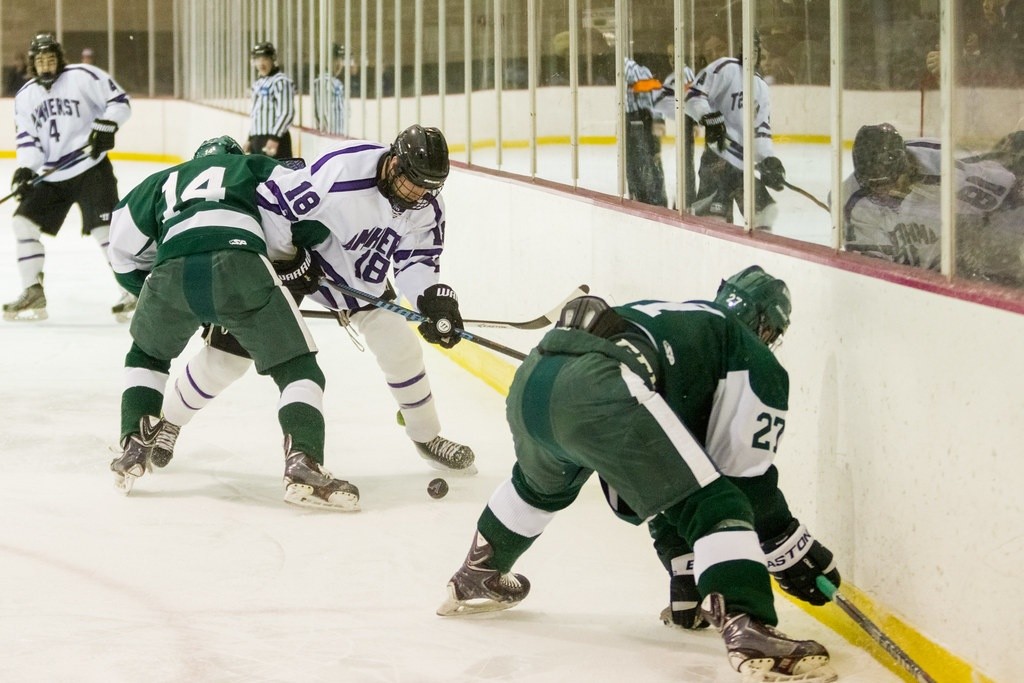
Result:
[414,283,464,350]
[699,111,728,153]
[760,155,786,191]
[271,248,326,296]
[89,121,115,158]
[11,171,40,201]
[759,518,841,605]
[670,554,703,630]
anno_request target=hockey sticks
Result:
[298,284,590,331]
[0,144,93,205]
[319,277,529,362]
[815,575,938,683]
[723,135,831,213]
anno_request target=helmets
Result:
[715,267,793,351]
[753,25,761,72]
[382,127,447,209]
[253,42,277,60]
[852,122,917,207]
[332,43,351,59]
[27,33,64,82]
[193,135,245,159]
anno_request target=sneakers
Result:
[113,289,138,323]
[700,592,839,683]
[153,421,181,468]
[109,414,163,495]
[3,284,49,323]
[278,434,360,514]
[437,529,530,616]
[414,437,477,478]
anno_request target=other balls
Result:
[427,478,449,500]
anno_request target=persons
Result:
[8,46,101,100]
[0,31,138,321]
[242,42,295,159]
[108,135,362,513]
[312,42,348,138]
[550,1,1024,292]
[437,265,841,682]
[146,124,478,476]
